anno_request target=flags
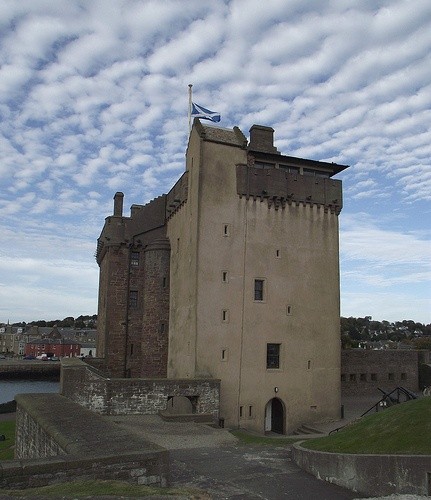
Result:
[190,101,221,124]
